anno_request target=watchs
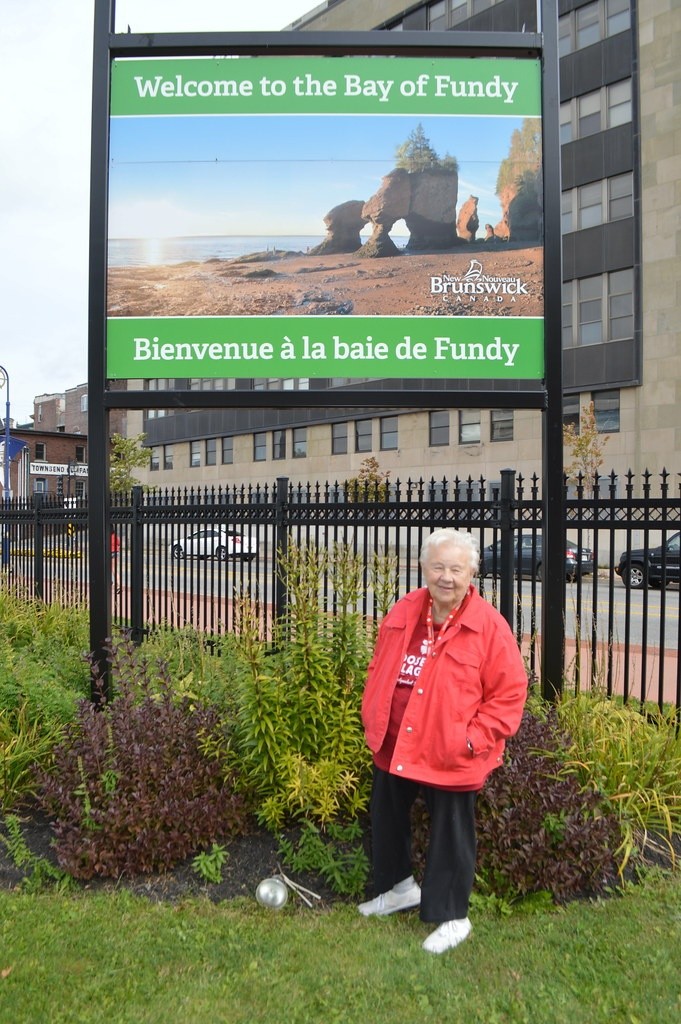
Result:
[467,739,472,750]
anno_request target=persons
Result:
[356,529,528,956]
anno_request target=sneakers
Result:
[423,917,472,954]
[357,884,421,917]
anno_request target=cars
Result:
[473,534,595,585]
[614,531,681,590]
[168,529,257,562]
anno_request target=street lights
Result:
[67,456,79,496]
